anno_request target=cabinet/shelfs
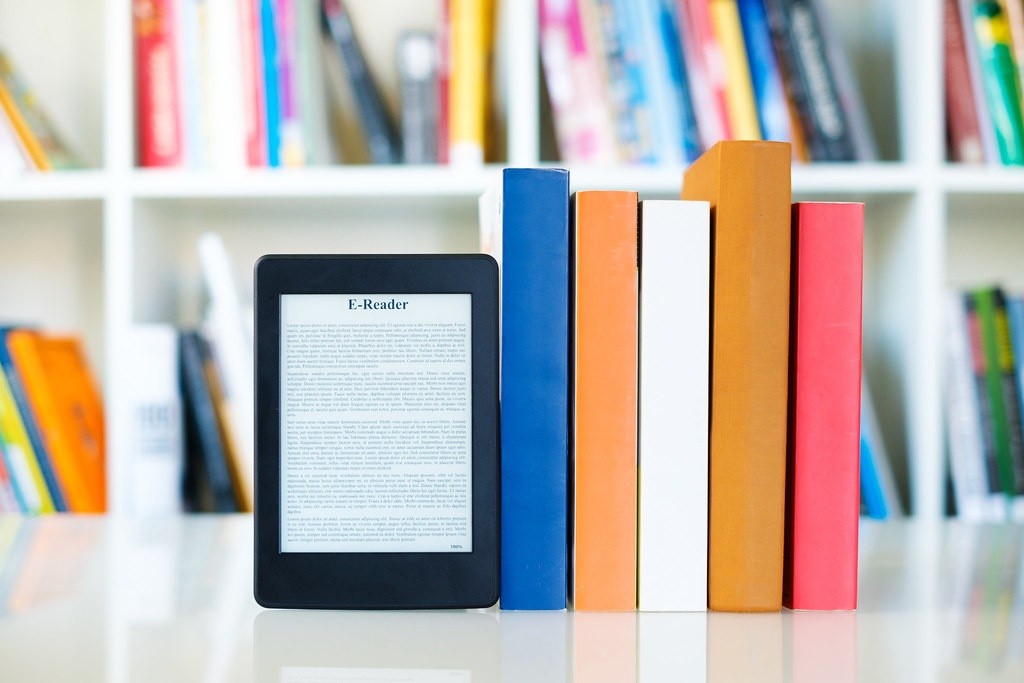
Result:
[0,1,511,520]
[509,1,1024,520]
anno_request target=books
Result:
[537,0,1023,172]
[140,226,257,516]
[858,278,1024,526]
[0,52,107,514]
[131,0,492,169]
[478,139,869,613]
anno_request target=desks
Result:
[4,504,1024,681]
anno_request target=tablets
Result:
[253,254,501,610]
